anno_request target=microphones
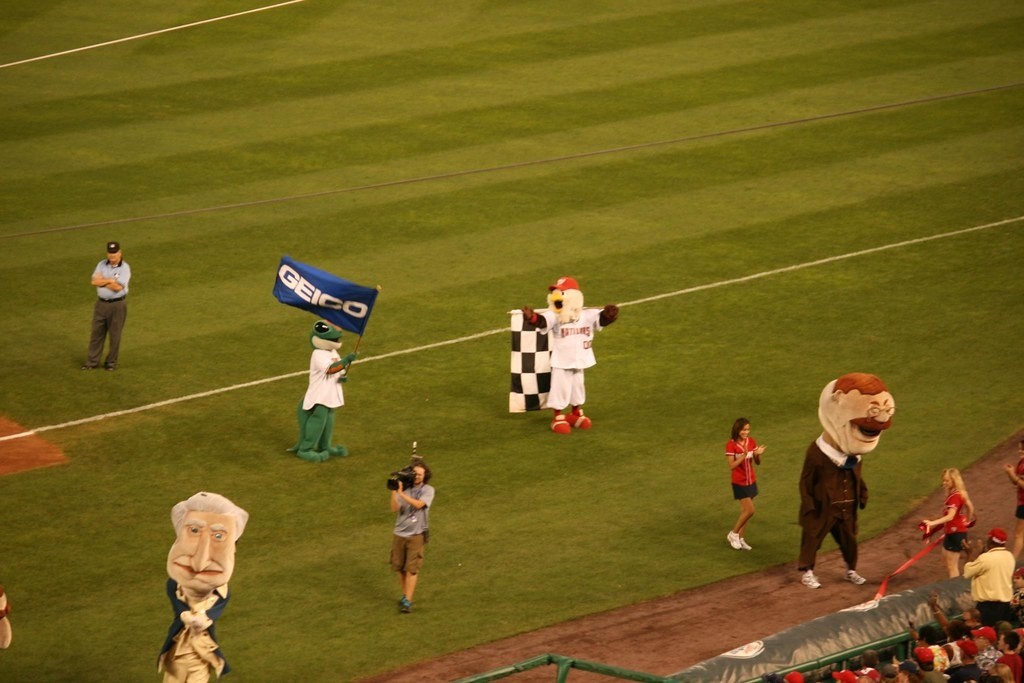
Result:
[391,471,399,475]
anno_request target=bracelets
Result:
[1015,478,1021,484]
[932,609,940,615]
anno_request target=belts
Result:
[99,296,125,303]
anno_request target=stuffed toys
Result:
[798,372,896,589]
[157,491,249,683]
[0,585,13,649]
[286,319,359,461]
[522,276,619,434]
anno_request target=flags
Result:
[507,309,554,413]
[273,256,382,336]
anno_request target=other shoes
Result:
[107,367,113,371]
[81,363,97,370]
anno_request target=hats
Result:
[831,670,858,683]
[883,665,898,679]
[914,646,934,664]
[1012,567,1024,577]
[899,661,917,674]
[855,667,882,683]
[957,640,978,658]
[987,528,1007,545]
[107,241,120,252]
[971,626,997,644]
[784,672,805,683]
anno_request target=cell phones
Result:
[909,622,915,628]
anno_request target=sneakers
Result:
[401,600,412,613]
[740,538,753,551]
[801,570,822,589]
[400,596,406,605]
[726,531,742,550]
[843,570,867,586]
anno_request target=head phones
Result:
[422,465,431,481]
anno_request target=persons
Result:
[961,528,1015,628]
[922,467,974,579]
[390,458,435,613]
[1003,439,1024,561]
[761,567,1024,683]
[81,241,131,370]
[726,417,768,552]
